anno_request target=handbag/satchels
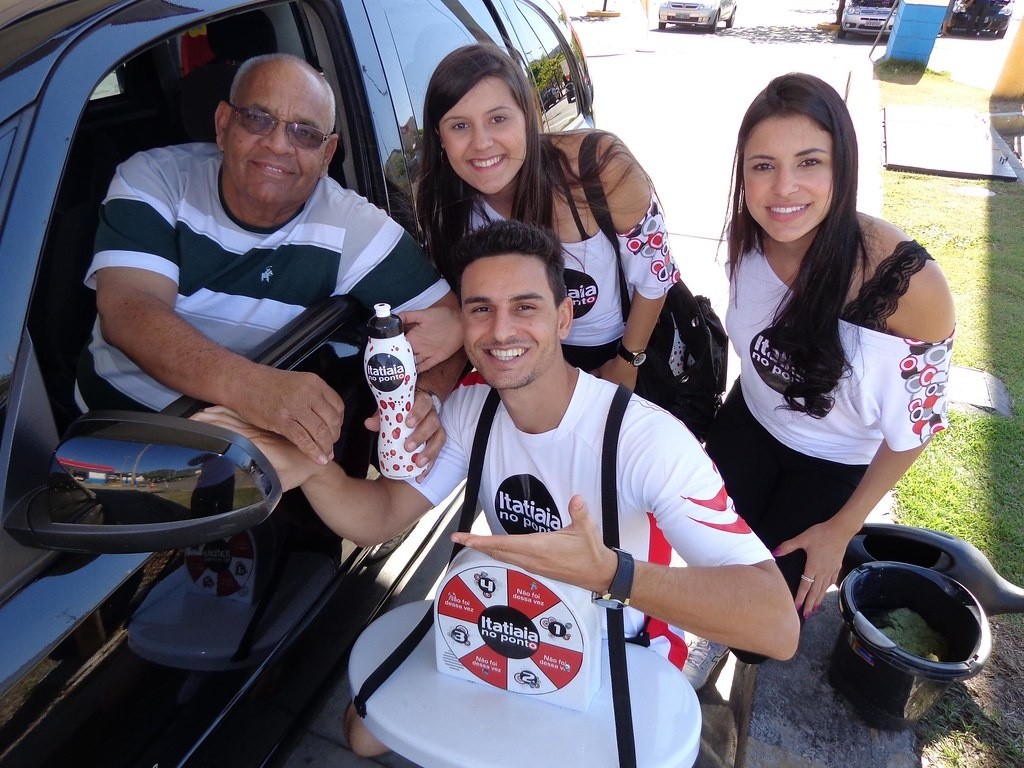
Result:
[641,279,729,445]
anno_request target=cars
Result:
[0,0,598,768]
[658,0,738,34]
[837,0,900,39]
[948,0,1015,38]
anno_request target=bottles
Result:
[363,303,428,480]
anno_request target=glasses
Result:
[226,101,330,151]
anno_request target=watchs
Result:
[591,546,634,610]
[415,387,444,417]
[616,340,648,367]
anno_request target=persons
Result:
[395,43,681,415]
[681,72,956,693]
[65,51,475,485]
[188,219,803,768]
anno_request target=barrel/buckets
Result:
[828,560,993,730]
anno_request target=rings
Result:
[414,355,417,364]
[801,575,815,583]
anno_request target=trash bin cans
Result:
[884,1,950,70]
